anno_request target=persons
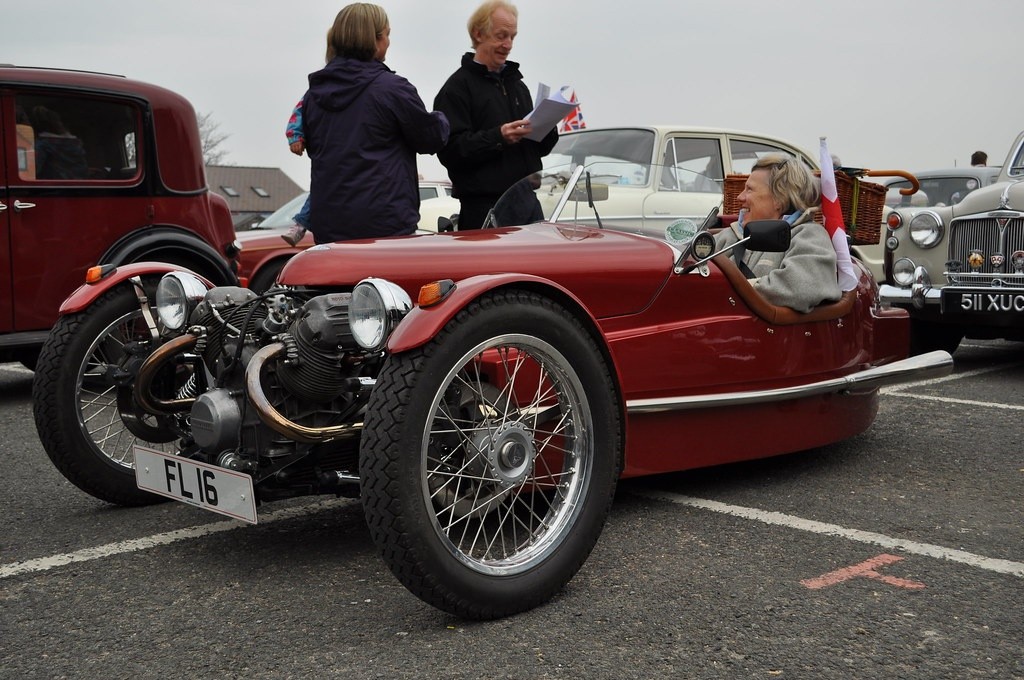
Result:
[434,0,559,231]
[711,152,842,311]
[970,151,987,167]
[280,2,449,248]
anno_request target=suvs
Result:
[0,61,243,393]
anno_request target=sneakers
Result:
[280,223,306,248]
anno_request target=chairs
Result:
[695,170,723,193]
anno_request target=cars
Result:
[233,180,453,325]
[881,165,1006,209]
[33,162,956,627]
[416,124,891,290]
[881,128,1023,363]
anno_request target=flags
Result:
[817,146,858,292]
[560,94,586,132]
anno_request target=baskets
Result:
[724,173,889,245]
